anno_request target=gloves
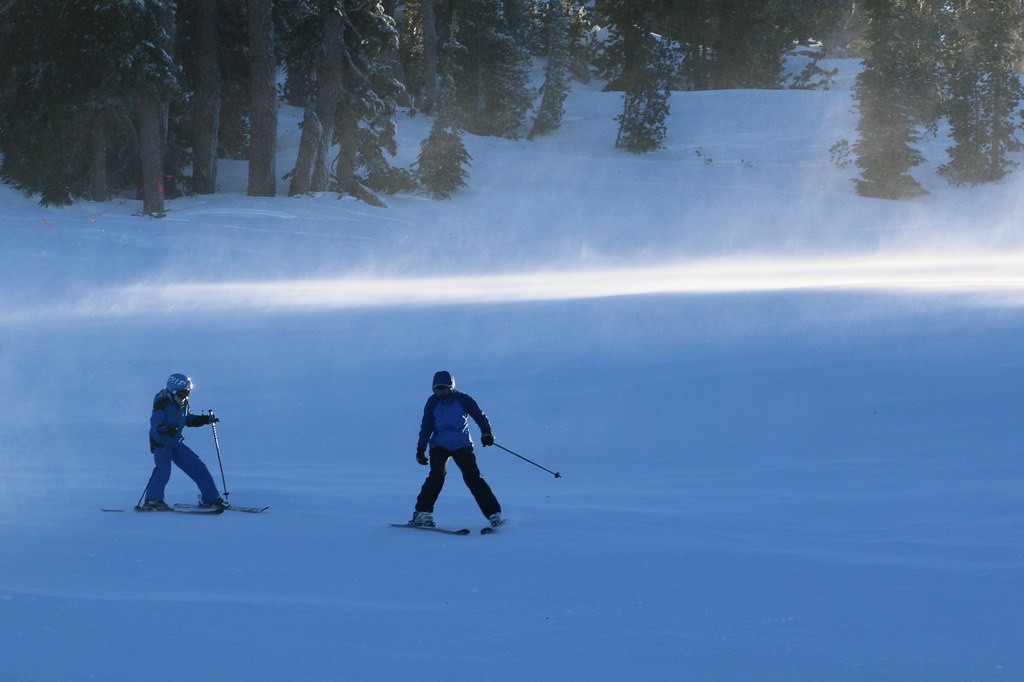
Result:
[480,432,495,447]
[416,449,428,465]
[170,426,178,436]
[208,414,219,425]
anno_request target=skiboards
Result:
[100,503,270,516]
[388,517,510,536]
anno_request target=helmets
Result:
[167,373,193,407]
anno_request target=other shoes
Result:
[198,496,230,508]
[489,512,506,528]
[412,512,436,527]
[143,499,168,508]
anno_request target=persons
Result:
[144,373,231,508]
[412,371,502,528]
